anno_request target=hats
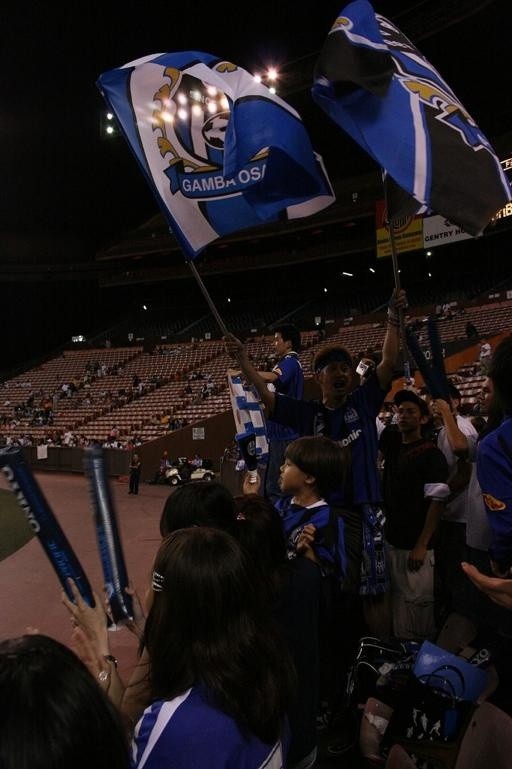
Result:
[394,389,428,420]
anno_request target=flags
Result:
[311,0,512,238]
[97,51,335,261]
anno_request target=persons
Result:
[1,287,510,767]
